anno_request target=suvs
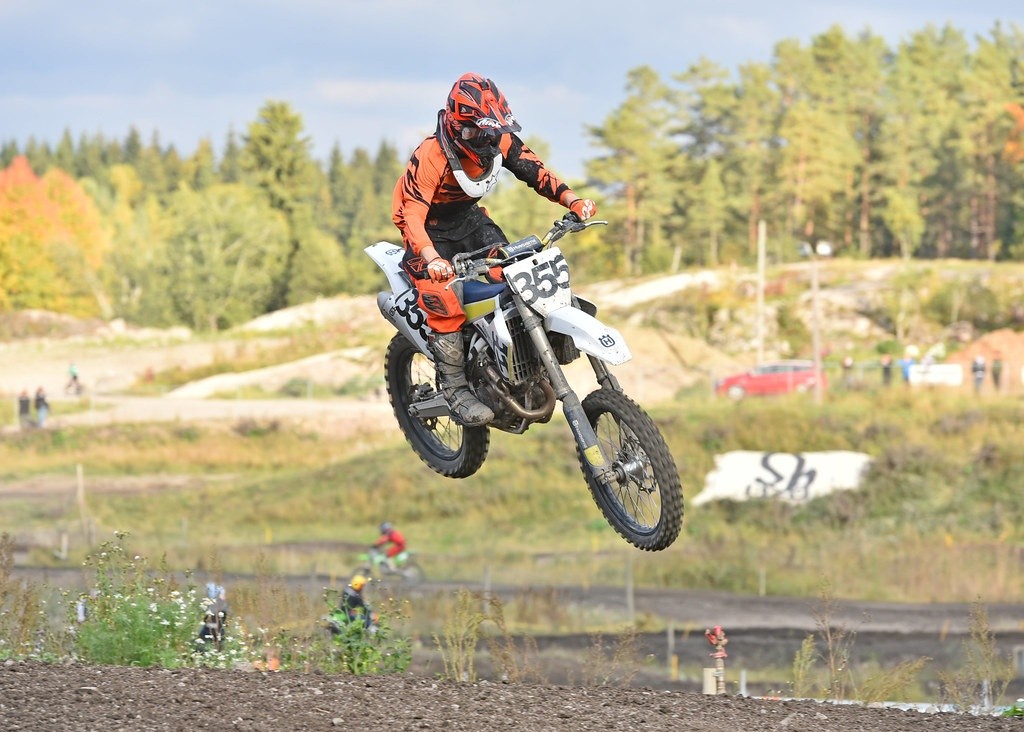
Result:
[717,362,827,400]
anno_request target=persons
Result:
[392,73,596,426]
[35,387,49,429]
[64,361,80,392]
[18,389,30,427]
[371,522,406,573]
[841,351,1003,395]
[340,575,370,631]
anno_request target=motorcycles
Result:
[362,213,684,552]
[352,553,424,589]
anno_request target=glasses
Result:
[462,126,503,147]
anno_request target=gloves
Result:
[571,199,597,221]
[426,258,454,283]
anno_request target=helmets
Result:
[445,74,521,171]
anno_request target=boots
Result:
[429,330,495,427]
